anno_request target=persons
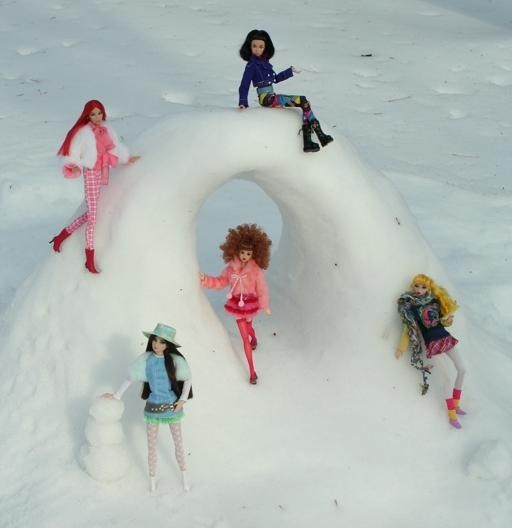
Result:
[199,224,273,385]
[48,99,142,276]
[102,322,193,494]
[394,274,467,430]
[238,29,333,152]
